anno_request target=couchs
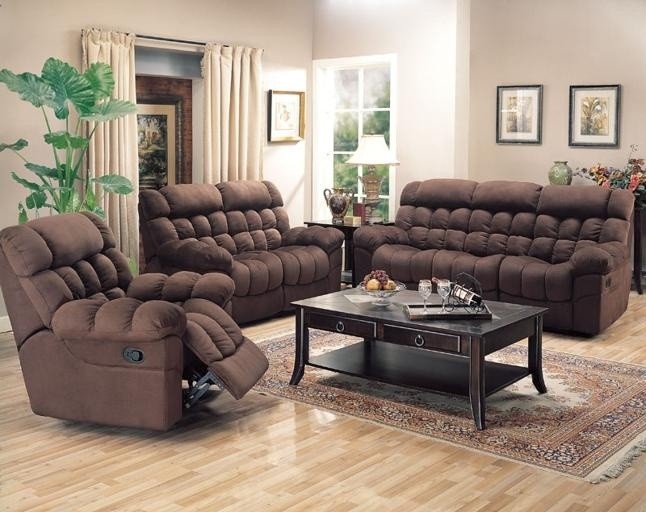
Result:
[352,178,636,336]
[138,178,346,326]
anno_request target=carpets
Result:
[249,328,646,486]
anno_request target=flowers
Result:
[568,143,646,204]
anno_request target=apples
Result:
[384,280,397,290]
[366,279,381,291]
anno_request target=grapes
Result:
[363,270,389,291]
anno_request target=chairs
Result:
[0,211,270,434]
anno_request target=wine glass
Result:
[435,279,452,314]
[358,279,406,306]
[418,279,433,312]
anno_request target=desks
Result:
[303,219,385,283]
[633,204,646,294]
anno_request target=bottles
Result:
[431,277,483,308]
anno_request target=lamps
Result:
[344,133,401,224]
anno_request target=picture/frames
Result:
[496,84,543,144]
[568,84,621,149]
[267,89,306,143]
[135,97,184,196]
